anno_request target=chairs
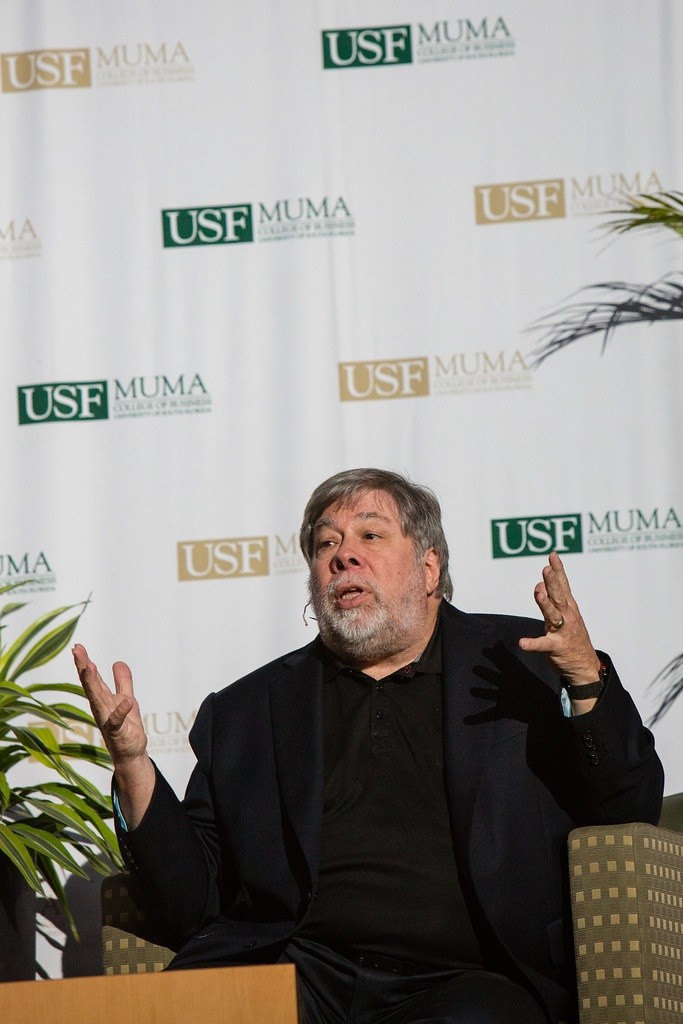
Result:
[98,794,683,1024]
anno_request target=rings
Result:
[553,616,565,630]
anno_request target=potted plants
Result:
[0,578,127,983]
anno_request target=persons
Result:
[70,468,666,1024]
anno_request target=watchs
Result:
[568,665,607,702]
